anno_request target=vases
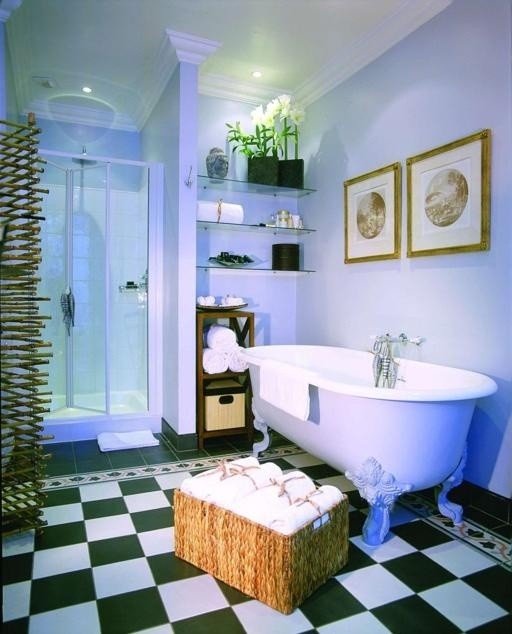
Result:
[247,155,278,189]
[278,159,309,189]
[271,242,301,270]
[204,146,229,179]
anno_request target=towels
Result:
[197,296,215,306]
[221,297,242,306]
[259,362,315,422]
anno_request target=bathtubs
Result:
[237,345,498,546]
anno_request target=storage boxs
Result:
[204,379,246,432]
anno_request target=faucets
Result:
[371,331,426,355]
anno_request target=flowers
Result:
[225,96,279,157]
[277,94,304,159]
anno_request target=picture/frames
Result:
[344,161,402,264]
[405,128,493,258]
